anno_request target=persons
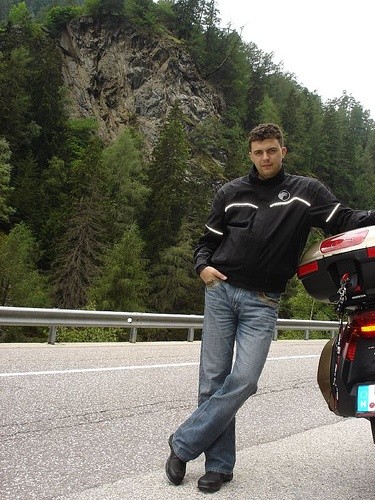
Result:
[166,124,375,492]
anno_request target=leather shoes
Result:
[165,433,186,485]
[198,471,233,492]
